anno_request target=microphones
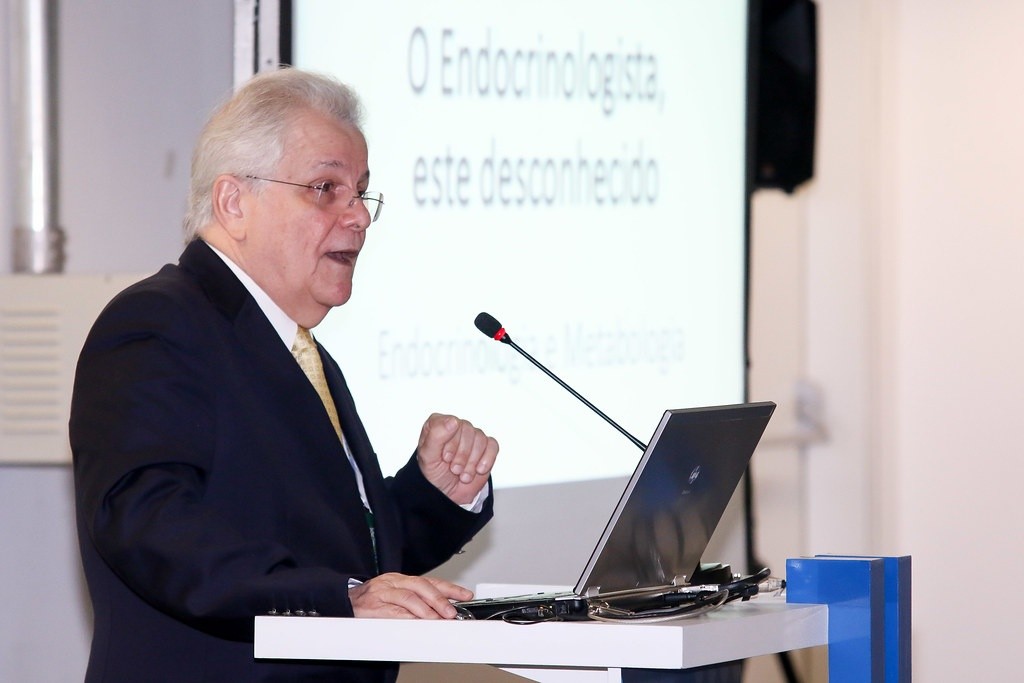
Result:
[474,313,647,454]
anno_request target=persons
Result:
[67,67,498,683]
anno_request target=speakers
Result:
[754,0,818,195]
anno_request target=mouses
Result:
[452,607,474,621]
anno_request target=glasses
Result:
[231,173,384,223]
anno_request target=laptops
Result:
[456,401,777,619]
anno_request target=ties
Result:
[292,325,343,443]
